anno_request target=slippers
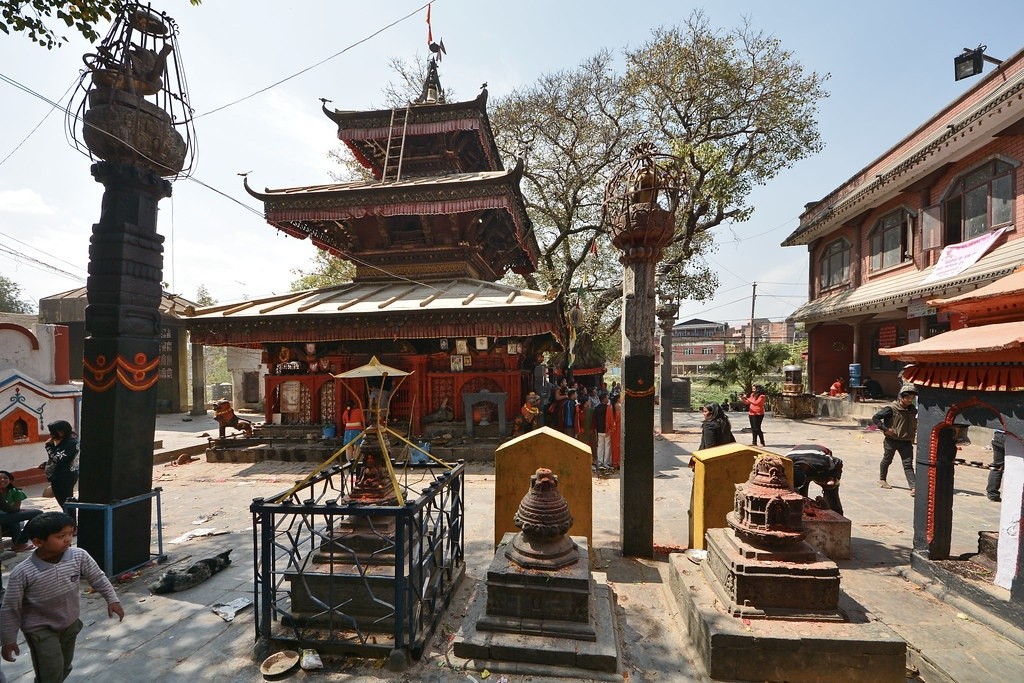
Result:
[15,544,36,552]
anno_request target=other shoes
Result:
[911,489,916,495]
[877,480,892,489]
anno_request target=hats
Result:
[901,383,918,396]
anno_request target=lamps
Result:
[954,44,987,81]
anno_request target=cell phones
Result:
[52,432,59,439]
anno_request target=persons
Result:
[721,393,750,412]
[872,385,917,497]
[547,378,621,475]
[342,400,366,462]
[860,376,883,399]
[0,470,43,550]
[738,384,765,446]
[784,444,843,516]
[830,376,847,396]
[370,382,393,423]
[698,401,736,451]
[986,430,1006,502]
[45,420,80,536]
[0,512,125,683]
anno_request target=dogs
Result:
[148,548,234,594]
